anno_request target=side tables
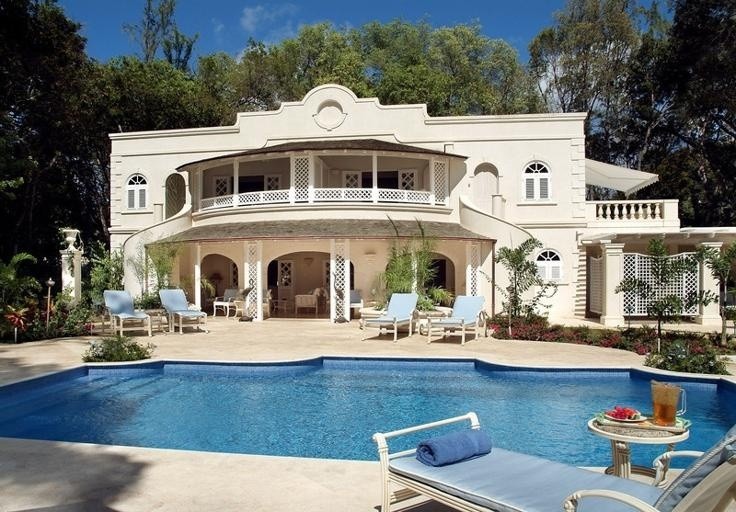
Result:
[588,411,690,486]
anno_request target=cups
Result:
[651,383,687,426]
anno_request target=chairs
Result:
[362,293,487,345]
[103,288,251,337]
[373,411,736,512]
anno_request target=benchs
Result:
[295,294,318,315]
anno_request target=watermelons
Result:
[607,406,640,420]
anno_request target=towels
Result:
[417,428,493,466]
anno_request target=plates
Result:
[604,414,647,422]
